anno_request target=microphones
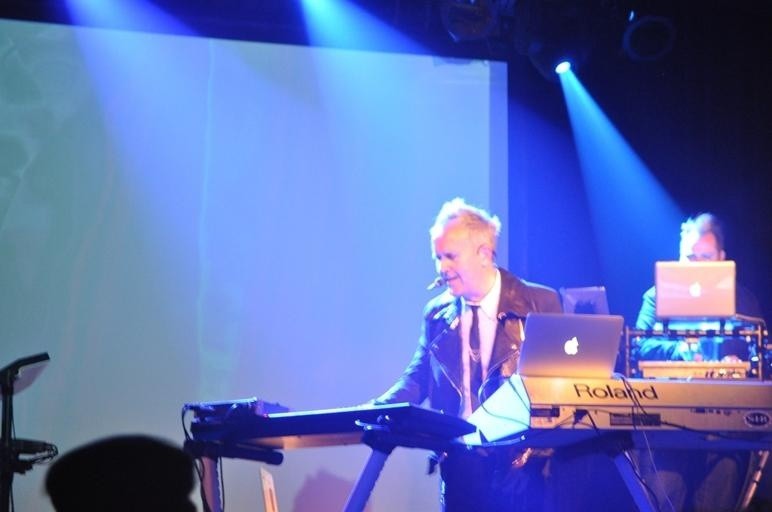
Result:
[428,276,446,290]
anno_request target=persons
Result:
[367,197,564,512]
[631,213,766,512]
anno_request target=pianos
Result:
[191,401,476,450]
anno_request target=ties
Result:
[468,305,483,413]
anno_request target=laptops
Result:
[516,313,624,379]
[654,261,765,322]
